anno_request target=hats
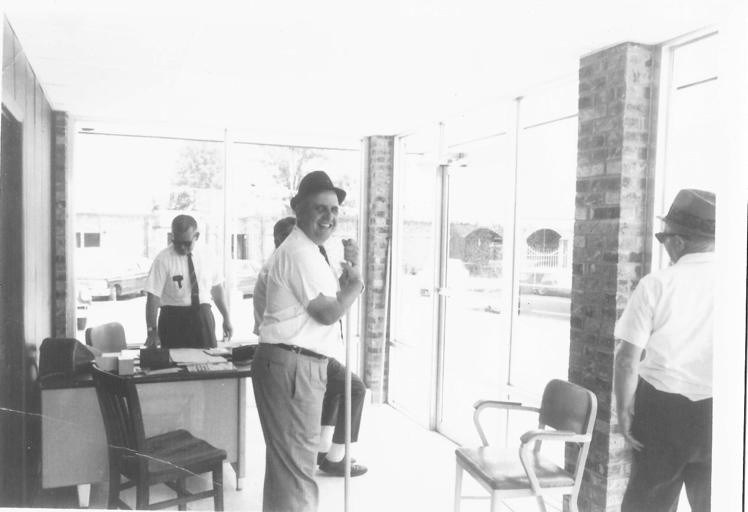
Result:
[290,170,346,209]
[655,188,716,241]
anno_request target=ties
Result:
[186,251,202,314]
[318,243,331,263]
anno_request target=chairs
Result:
[85,321,127,354]
[453,378,598,512]
[89,359,228,511]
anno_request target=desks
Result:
[40,347,252,507]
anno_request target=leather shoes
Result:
[319,452,368,479]
[316,451,326,465]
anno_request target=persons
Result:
[612,187,716,512]
[249,170,363,512]
[267,217,369,479]
[142,215,235,349]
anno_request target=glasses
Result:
[655,231,691,243]
[171,234,198,248]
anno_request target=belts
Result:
[277,342,328,361]
